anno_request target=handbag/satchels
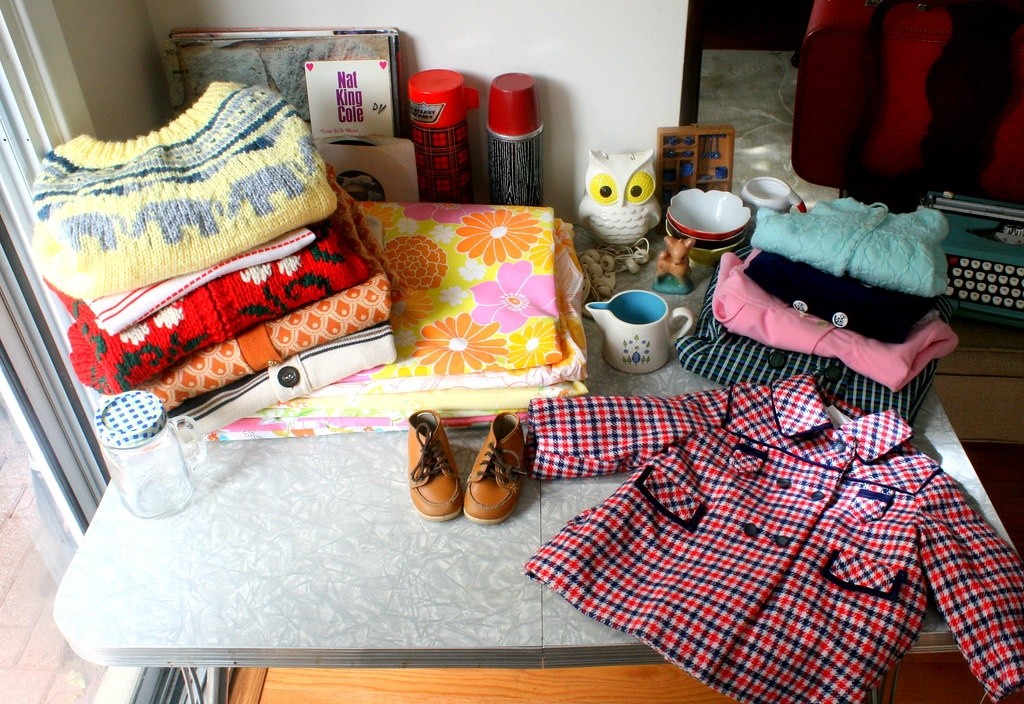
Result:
[791,0,1024,199]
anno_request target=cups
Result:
[585,289,693,374]
[95,389,206,521]
[406,69,481,204]
[741,177,807,241]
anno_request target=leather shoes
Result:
[463,412,526,524]
[408,410,462,522]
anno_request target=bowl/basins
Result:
[665,188,751,266]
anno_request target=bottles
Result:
[484,73,545,207]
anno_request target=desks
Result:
[52,205,1024,704]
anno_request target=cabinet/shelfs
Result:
[656,124,735,229]
